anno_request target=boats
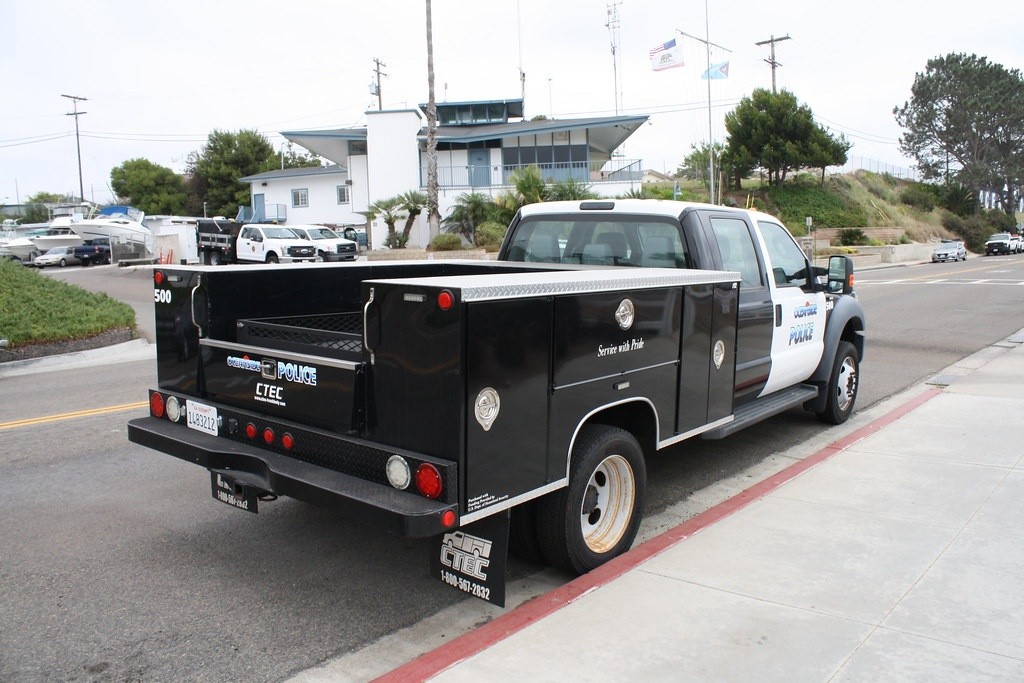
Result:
[0,219,36,261]
[69,204,153,245]
[28,216,83,255]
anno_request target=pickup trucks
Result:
[985,234,1019,256]
[126,198,867,609]
[285,224,359,262]
[197,222,319,264]
[66,238,118,267]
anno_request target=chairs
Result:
[596,233,627,265]
[583,244,615,265]
[715,236,732,262]
[530,233,561,263]
[642,236,677,268]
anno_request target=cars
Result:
[932,240,967,263]
[33,246,82,269]
[1011,236,1024,253]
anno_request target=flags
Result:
[649,39,685,71]
[701,59,730,80]
